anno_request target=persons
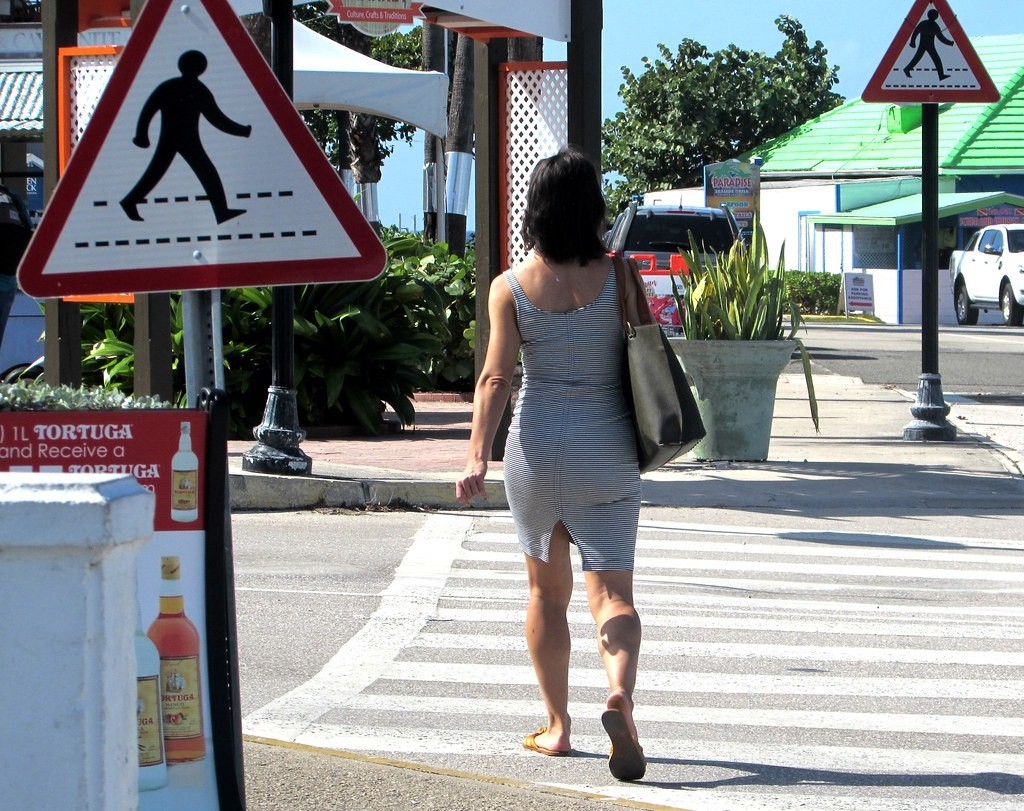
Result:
[453,148,655,781]
[0,182,34,347]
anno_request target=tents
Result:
[293,12,448,242]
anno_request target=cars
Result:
[0,184,46,389]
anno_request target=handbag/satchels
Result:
[612,254,707,473]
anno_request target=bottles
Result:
[169,421,199,523]
[147,554,206,763]
[133,591,170,793]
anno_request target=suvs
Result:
[948,223,1024,326]
[602,200,749,289]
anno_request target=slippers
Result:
[523,725,567,759]
[601,708,646,781]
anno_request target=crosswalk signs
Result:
[862,0,1001,102]
[16,0,392,295]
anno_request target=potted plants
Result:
[666,207,820,464]
